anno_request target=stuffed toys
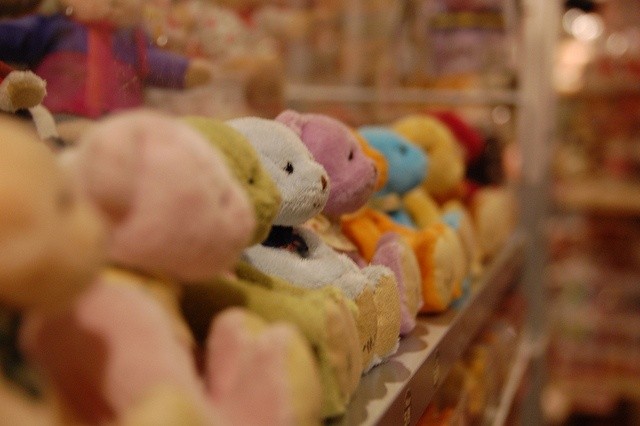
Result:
[1,72,515,426]
[0,72,515,426]
[0,2,284,118]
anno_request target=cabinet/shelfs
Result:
[4,4,542,426]
